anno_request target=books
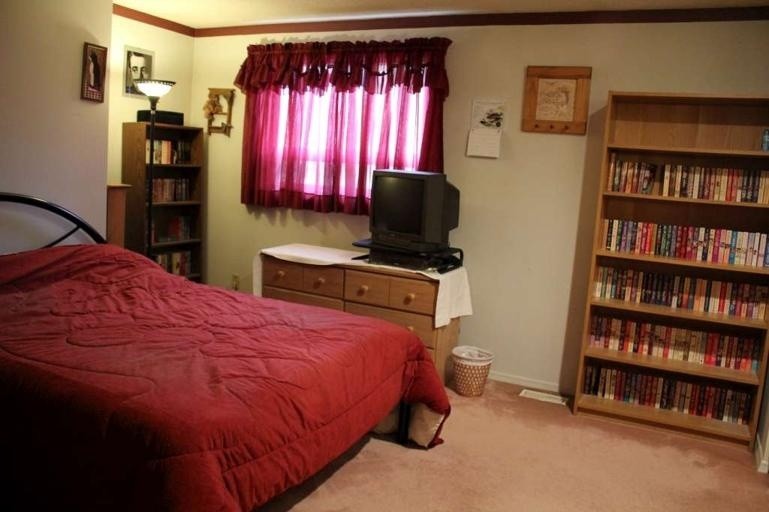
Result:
[601,219,769,268]
[156,214,191,240]
[589,317,762,375]
[151,177,191,201]
[145,138,186,166]
[156,251,193,276]
[584,364,753,425]
[608,153,769,205]
[593,265,768,322]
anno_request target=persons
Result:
[125,52,152,95]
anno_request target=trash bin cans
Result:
[452,345,493,397]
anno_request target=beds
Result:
[0,192,408,510]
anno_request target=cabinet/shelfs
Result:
[120,122,206,283]
[264,243,461,387]
[572,91,768,449]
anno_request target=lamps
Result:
[133,80,175,256]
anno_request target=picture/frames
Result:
[81,41,108,102]
[121,46,157,97]
[521,64,592,134]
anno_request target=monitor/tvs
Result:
[369,169,459,252]
